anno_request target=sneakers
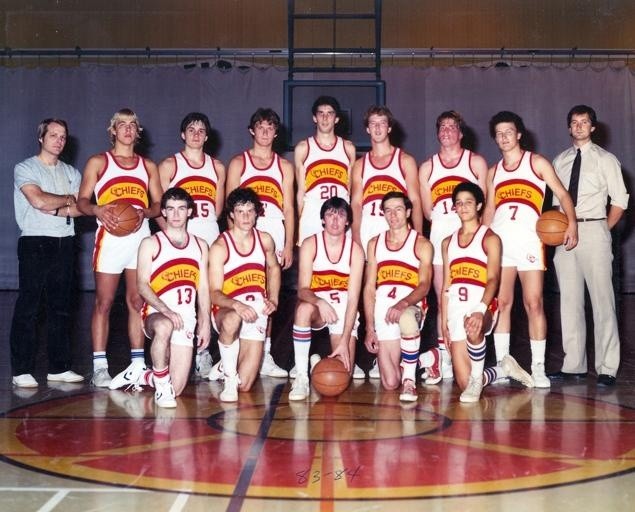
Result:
[259,355,289,379]
[90,368,113,388]
[194,351,213,379]
[307,353,382,380]
[399,378,419,402]
[420,347,453,385]
[46,369,85,383]
[459,373,484,403]
[108,361,147,391]
[209,358,224,381]
[500,354,552,389]
[153,384,177,409]
[11,373,39,389]
[288,373,312,401]
[219,378,240,403]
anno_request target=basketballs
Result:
[312,358,350,397]
[536,211,568,246]
[107,204,138,236]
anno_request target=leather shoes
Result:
[547,370,617,389]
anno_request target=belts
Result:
[576,217,608,223]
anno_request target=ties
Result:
[567,148,581,208]
